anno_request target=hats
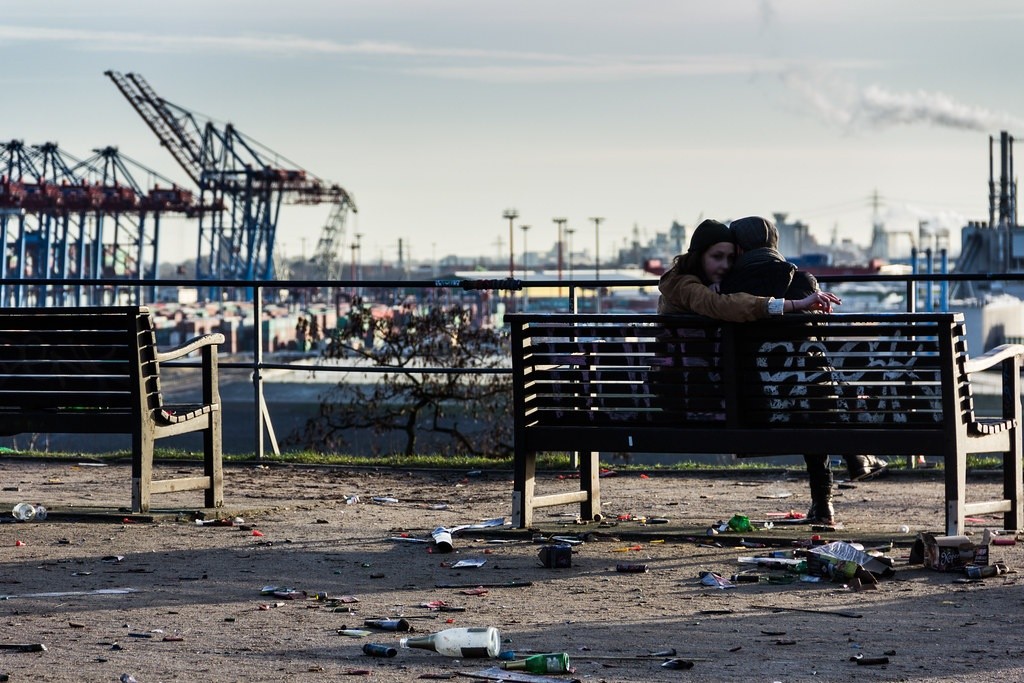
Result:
[730,216,779,252]
[688,219,736,256]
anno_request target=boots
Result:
[842,455,888,481]
[807,481,835,525]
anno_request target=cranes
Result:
[0,69,357,307]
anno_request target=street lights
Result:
[565,228,576,281]
[500,208,521,299]
[589,215,604,279]
[519,224,531,309]
[552,218,567,299]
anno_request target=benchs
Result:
[0,305,223,514]
[504,312,1024,536]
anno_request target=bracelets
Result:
[791,300,796,312]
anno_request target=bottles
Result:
[34,506,47,521]
[500,652,570,673]
[12,503,36,522]
[399,626,501,659]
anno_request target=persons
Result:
[716,216,889,524]
[649,215,842,422]
